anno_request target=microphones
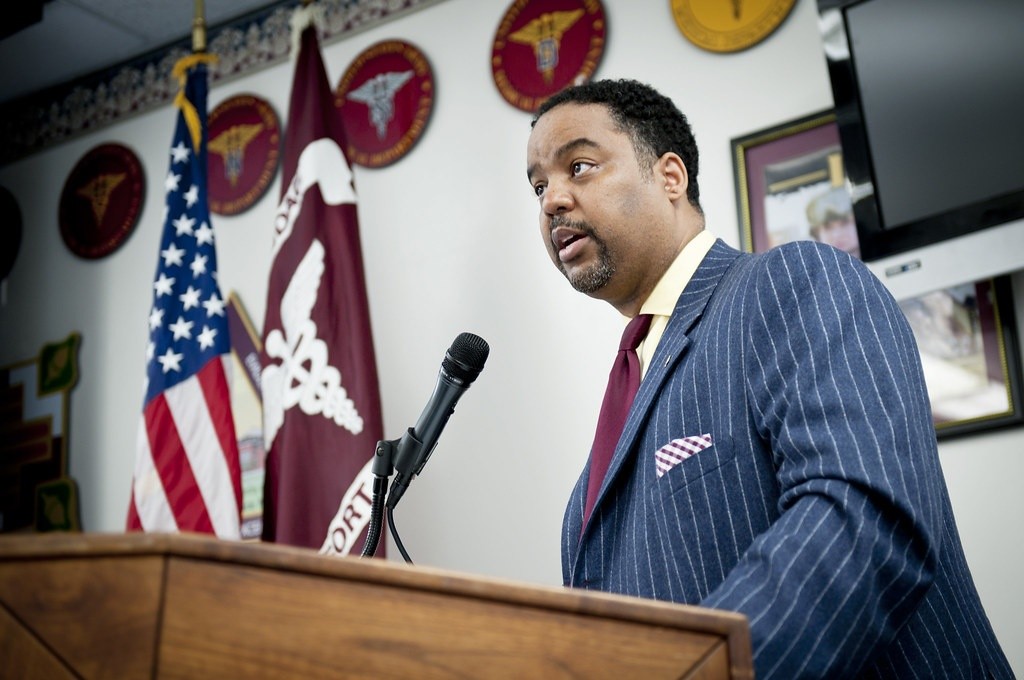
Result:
[386,331,490,510]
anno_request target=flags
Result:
[128,53,241,537]
[256,24,389,558]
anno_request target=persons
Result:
[807,187,1010,423]
[527,80,1016,679]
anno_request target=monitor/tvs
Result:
[817,0,1024,302]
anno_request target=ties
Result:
[577,314,654,542]
